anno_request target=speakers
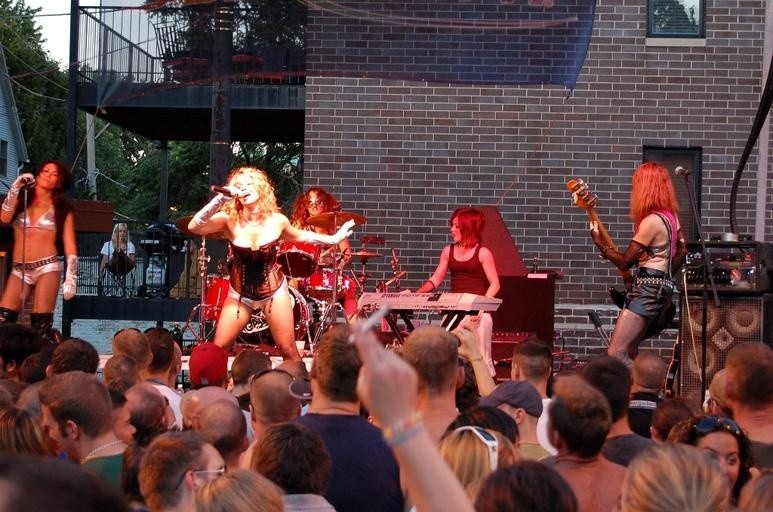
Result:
[678,293,772,404]
[640,144,703,242]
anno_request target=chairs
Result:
[142,18,306,84]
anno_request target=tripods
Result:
[179,249,219,345]
[311,245,352,350]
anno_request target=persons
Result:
[1,159,79,344]
[397,208,501,380]
[282,185,360,331]
[99,221,137,297]
[587,160,689,368]
[185,163,356,361]
[1,307,772,511]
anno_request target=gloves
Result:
[194,186,238,227]
[63,255,78,300]
[307,219,355,246]
[2,173,35,212]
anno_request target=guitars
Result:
[566,177,676,338]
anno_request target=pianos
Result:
[357,292,502,312]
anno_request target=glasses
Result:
[250,369,291,383]
[693,416,740,436]
[193,466,226,479]
[457,426,498,473]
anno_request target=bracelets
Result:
[598,245,614,261]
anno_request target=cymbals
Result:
[236,285,309,345]
[305,210,366,227]
[176,214,227,237]
[337,251,384,261]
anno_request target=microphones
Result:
[387,270,406,286]
[211,184,242,198]
[21,173,36,183]
[674,166,693,178]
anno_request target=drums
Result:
[276,240,319,277]
[202,272,232,322]
[302,267,344,299]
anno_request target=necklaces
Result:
[244,216,268,252]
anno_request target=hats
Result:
[189,342,228,385]
[480,380,542,417]
[288,381,312,399]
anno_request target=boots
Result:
[0,307,18,328]
[29,312,53,339]
